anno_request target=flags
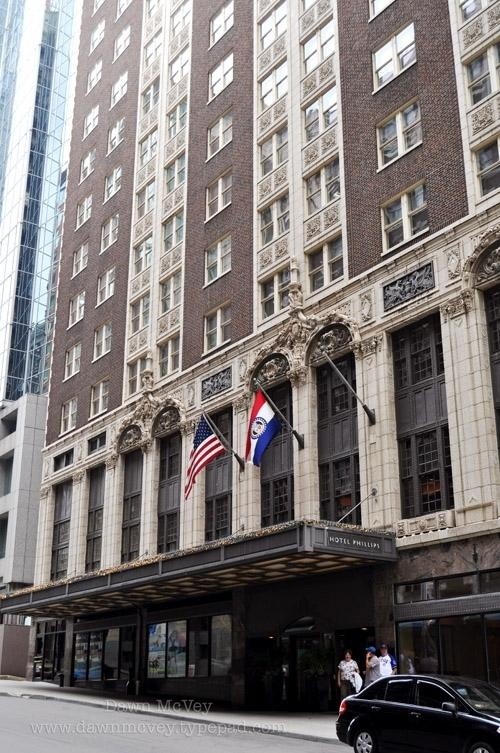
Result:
[183,413,224,500]
[245,389,281,467]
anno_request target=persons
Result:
[337,650,361,700]
[364,646,380,689]
[399,648,438,674]
[378,644,398,677]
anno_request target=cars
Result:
[33,655,57,680]
[335,674,500,753]
[72,661,114,681]
[54,672,63,684]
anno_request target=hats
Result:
[365,646,376,653]
